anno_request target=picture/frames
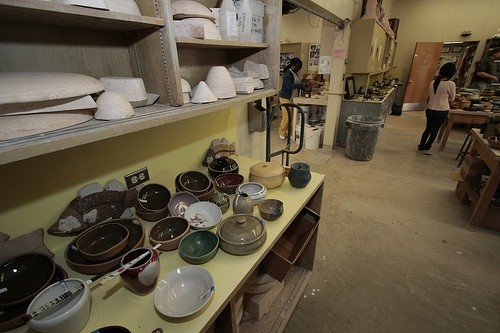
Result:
[346,76,356,100]
[441,46,451,53]
[451,46,463,53]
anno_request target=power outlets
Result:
[123,167,151,190]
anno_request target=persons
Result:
[417,62,456,155]
[279,57,311,139]
[469,47,500,90]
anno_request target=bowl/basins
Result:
[488,136,500,150]
[25,277,91,333]
[98,76,148,101]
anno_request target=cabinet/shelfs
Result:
[0,154,326,333]
[481,38,500,84]
[345,18,397,95]
[0,0,281,166]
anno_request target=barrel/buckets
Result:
[294,123,325,150]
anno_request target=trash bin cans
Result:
[346,116,385,161]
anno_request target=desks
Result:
[292,94,328,142]
[438,108,492,156]
[336,84,396,147]
[456,128,500,232]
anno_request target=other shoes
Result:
[280,134,285,139]
[425,150,432,155]
[418,149,426,154]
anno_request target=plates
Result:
[64,155,284,319]
[91,325,131,333]
[0,253,55,309]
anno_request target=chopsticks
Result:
[0,280,93,332]
[99,243,162,286]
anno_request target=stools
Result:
[455,130,474,168]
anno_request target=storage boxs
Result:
[449,168,500,232]
[260,207,320,283]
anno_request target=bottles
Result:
[237,0,253,42]
[288,162,312,188]
[120,248,159,297]
[219,0,238,42]
